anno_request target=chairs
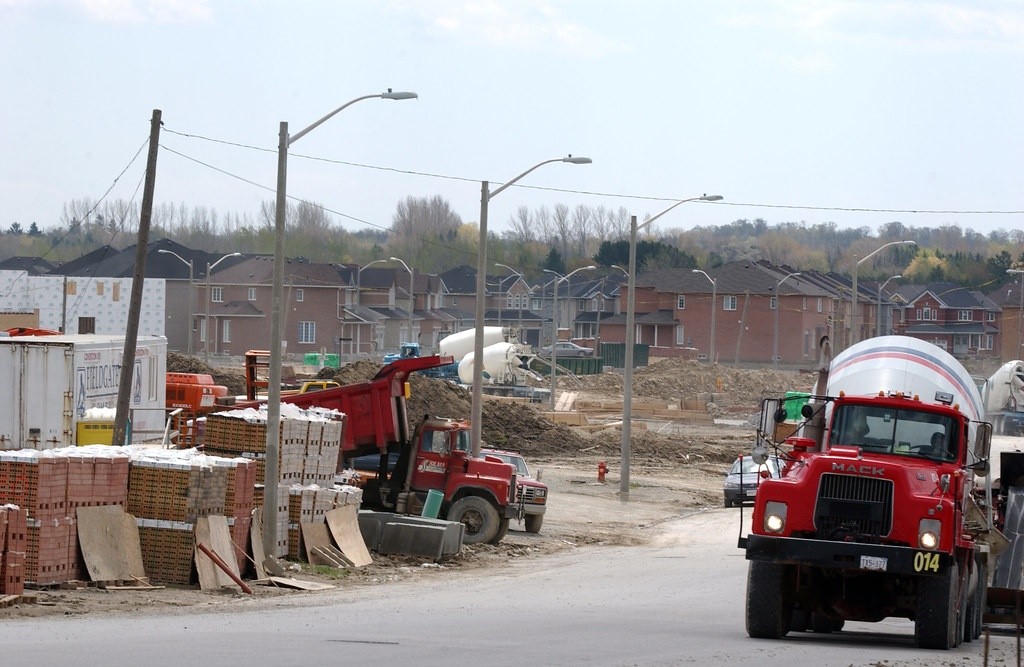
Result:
[916,432,951,462]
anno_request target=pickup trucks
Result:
[478,444,548,534]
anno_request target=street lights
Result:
[158,249,193,363]
[772,272,804,369]
[618,193,723,496]
[356,260,387,362]
[204,252,241,363]
[389,257,414,343]
[550,265,598,411]
[691,268,717,367]
[847,240,917,347]
[875,273,903,337]
[258,89,418,558]
[468,156,593,458]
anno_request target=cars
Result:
[721,448,787,511]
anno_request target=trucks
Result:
[181,354,524,545]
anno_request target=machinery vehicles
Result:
[726,335,1023,650]
[381,322,552,404]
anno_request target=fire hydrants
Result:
[596,460,609,482]
